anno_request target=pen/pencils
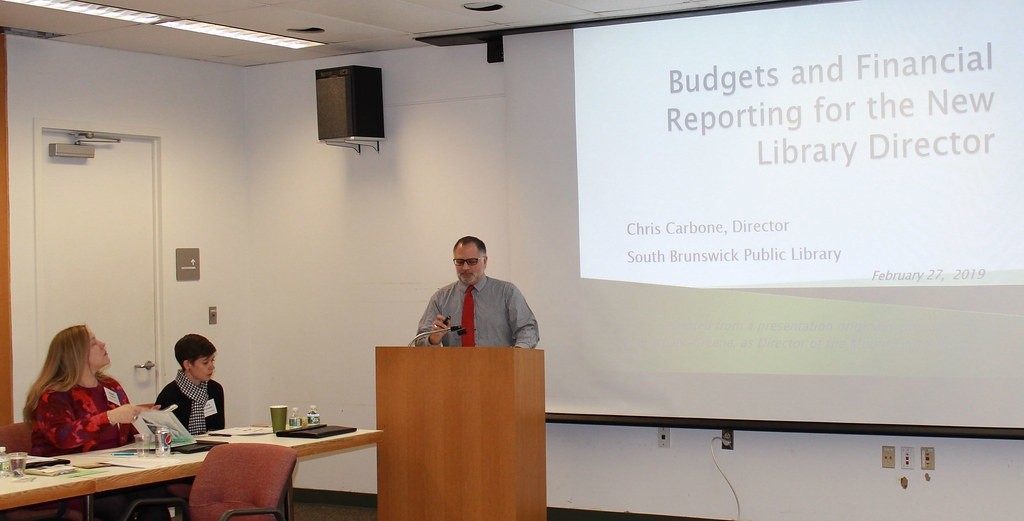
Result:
[208,434,232,436]
[111,453,137,456]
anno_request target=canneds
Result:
[155,428,171,457]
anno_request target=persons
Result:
[414,236,539,349]
[24,324,172,521]
[154,333,226,501]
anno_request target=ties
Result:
[462,286,474,347]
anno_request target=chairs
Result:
[0,404,297,521]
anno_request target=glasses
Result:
[453,257,484,266]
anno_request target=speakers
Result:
[315,65,384,140]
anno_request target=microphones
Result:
[407,325,466,348]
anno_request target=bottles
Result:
[306,406,320,427]
[288,407,303,430]
[0,446,12,482]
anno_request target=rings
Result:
[433,324,437,328]
[134,415,138,420]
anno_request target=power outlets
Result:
[882,446,896,468]
[920,447,935,470]
[721,430,734,450]
[901,447,913,469]
[658,427,670,449]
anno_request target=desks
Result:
[0,424,383,521]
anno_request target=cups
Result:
[8,452,28,481]
[134,433,151,457]
[270,405,287,433]
